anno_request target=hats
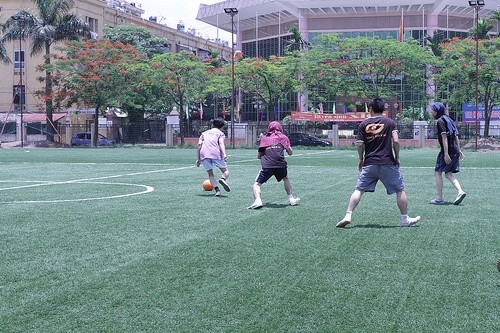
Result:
[432,102,445,120]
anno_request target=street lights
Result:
[468,0,486,145]
[11,14,26,148]
[224,8,239,149]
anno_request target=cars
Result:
[287,131,334,147]
[71,132,116,145]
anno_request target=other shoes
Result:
[219,179,230,192]
[401,216,421,227]
[430,197,445,204]
[247,198,263,209]
[291,198,301,206]
[214,190,221,196]
[453,191,466,205]
[336,215,352,227]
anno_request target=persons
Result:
[247,121,300,209]
[429,102,466,205]
[195,118,231,197]
[335,98,421,228]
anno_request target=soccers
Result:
[202,180,213,191]
